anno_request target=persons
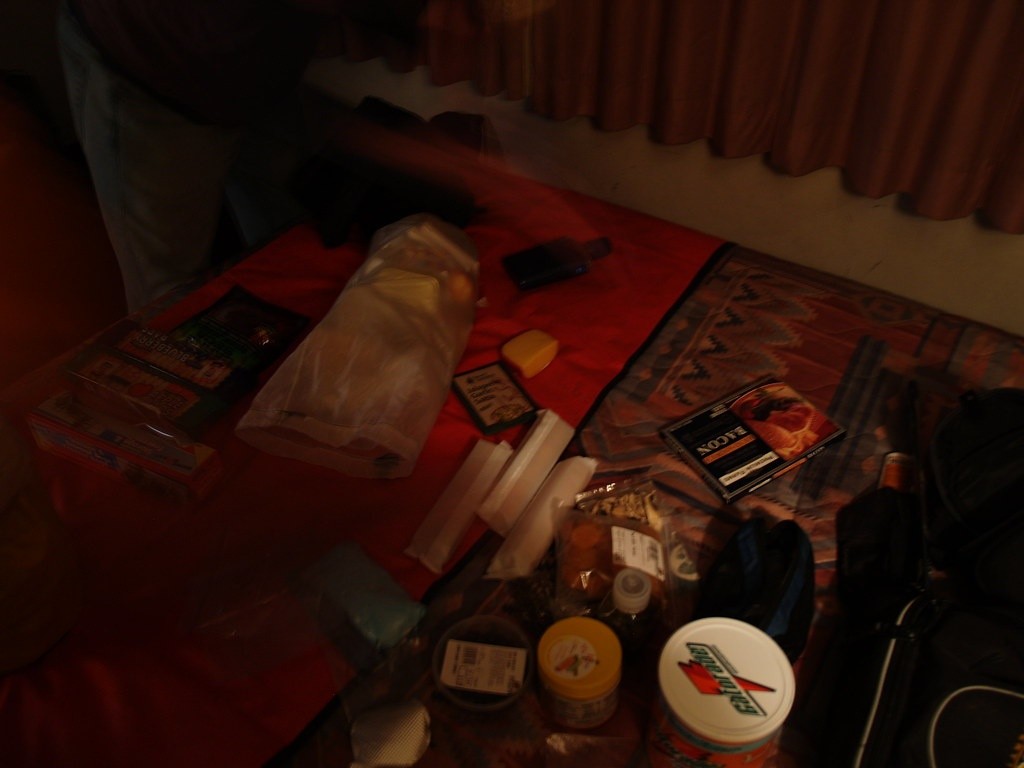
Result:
[56,0,588,314]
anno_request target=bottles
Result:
[613,570,652,655]
[646,618,796,768]
[538,617,621,727]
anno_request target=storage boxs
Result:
[29,316,254,514]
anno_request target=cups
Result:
[432,614,532,723]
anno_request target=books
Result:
[662,374,848,504]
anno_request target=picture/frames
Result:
[448,360,542,432]
